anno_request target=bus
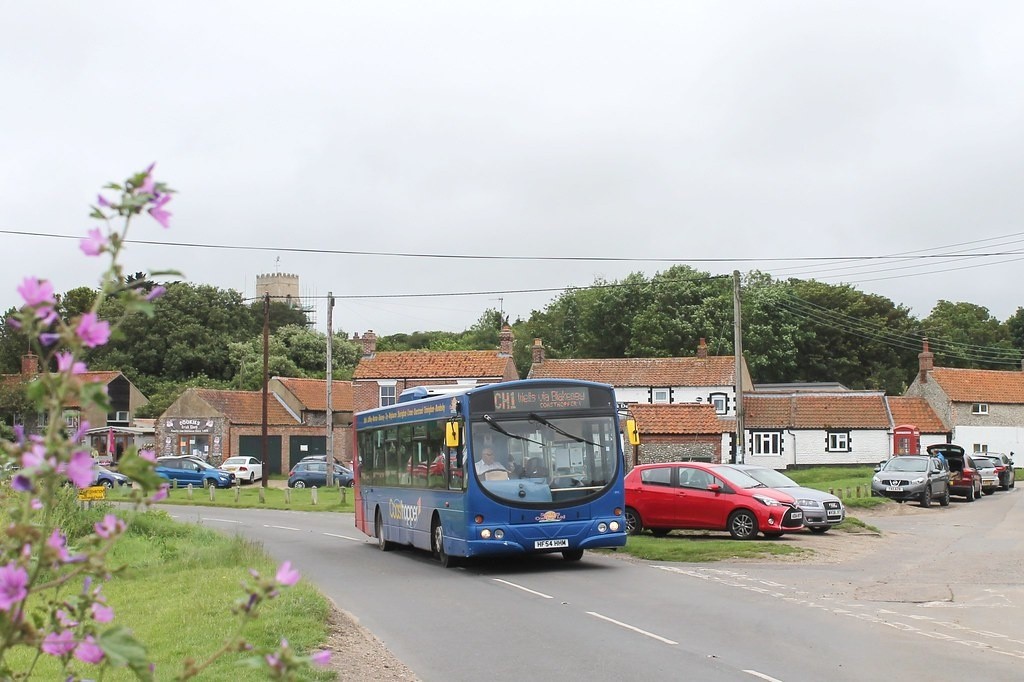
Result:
[353,377,640,568]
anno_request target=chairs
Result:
[688,470,707,489]
[679,471,688,484]
[648,469,669,483]
[186,462,194,470]
[527,458,545,478]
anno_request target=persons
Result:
[933,449,947,466]
[526,457,548,478]
[505,453,525,478]
[467,448,508,479]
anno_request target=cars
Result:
[219,456,262,484]
[289,455,354,488]
[719,464,846,534]
[622,463,803,540]
[59,463,133,490]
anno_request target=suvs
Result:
[153,455,234,490]
[927,443,983,502]
[968,451,1016,496]
[407,449,463,485]
[871,454,951,508]
